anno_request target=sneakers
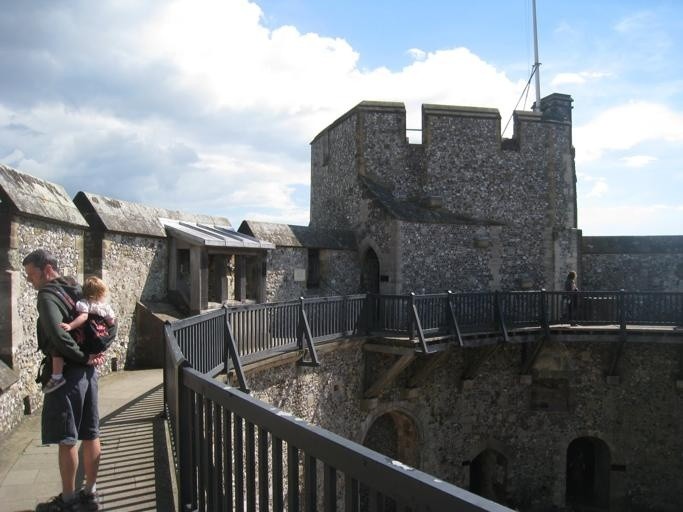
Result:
[42,375,67,395]
[35,493,83,512]
[80,488,100,512]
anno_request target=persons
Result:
[23,250,110,511]
[564,271,579,325]
[43,275,117,393]
